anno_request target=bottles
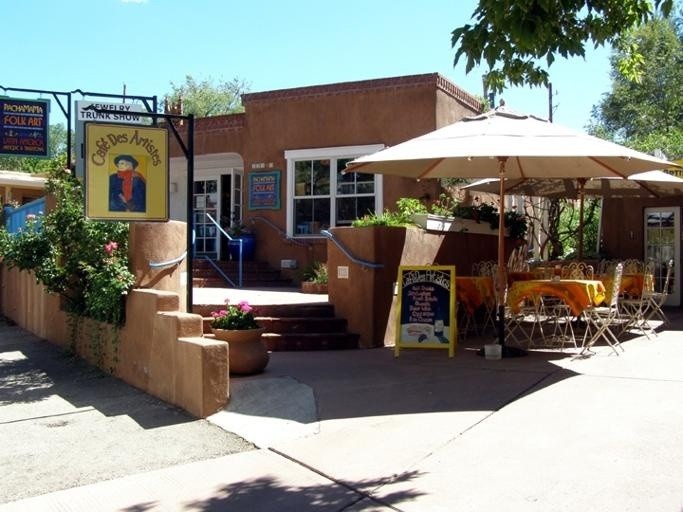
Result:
[554,264,561,281]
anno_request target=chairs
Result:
[456,255,674,364]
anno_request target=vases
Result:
[228,233,256,259]
[212,328,270,379]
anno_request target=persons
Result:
[109,155,146,212]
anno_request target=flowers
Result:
[222,216,257,236]
[209,298,260,329]
[429,191,529,250]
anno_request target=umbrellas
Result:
[459,170,683,275]
[341,98,683,358]
